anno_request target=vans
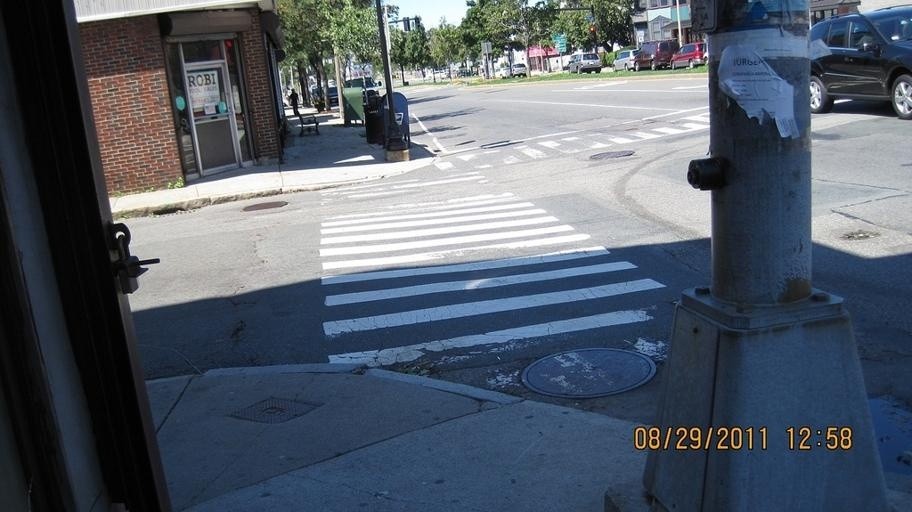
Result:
[345,77,383,102]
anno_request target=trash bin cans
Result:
[342,87,410,150]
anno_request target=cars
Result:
[428,59,485,81]
[307,80,340,109]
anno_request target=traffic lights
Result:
[415,17,421,31]
[590,25,595,35]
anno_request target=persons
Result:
[288,89,299,116]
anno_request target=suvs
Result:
[809,3,911,120]
[612,40,708,72]
[567,52,602,75]
[495,64,527,80]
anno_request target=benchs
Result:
[294,111,320,136]
